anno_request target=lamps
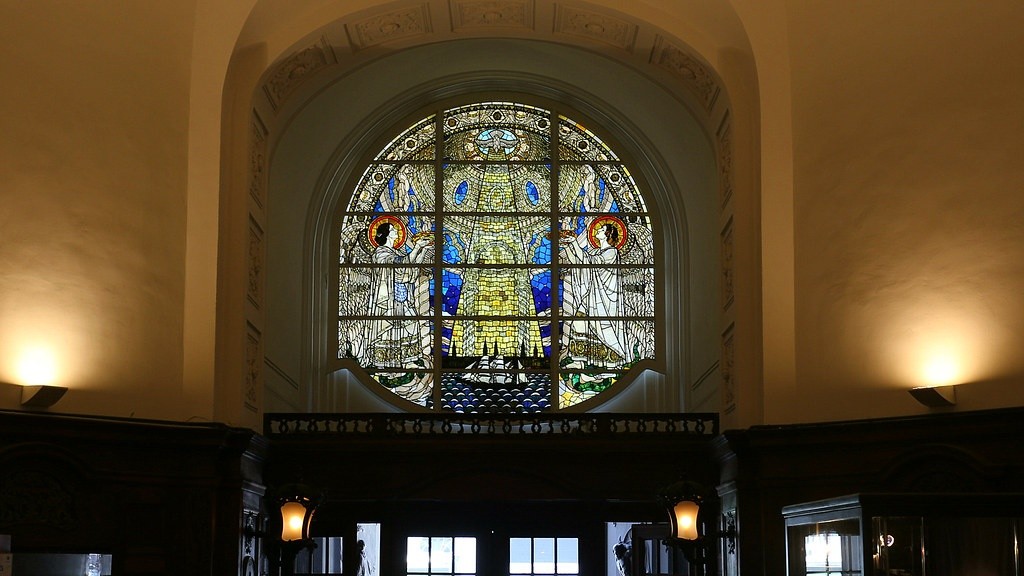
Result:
[650,465,736,566]
[244,464,326,569]
[907,384,956,410]
[21,385,68,408]
